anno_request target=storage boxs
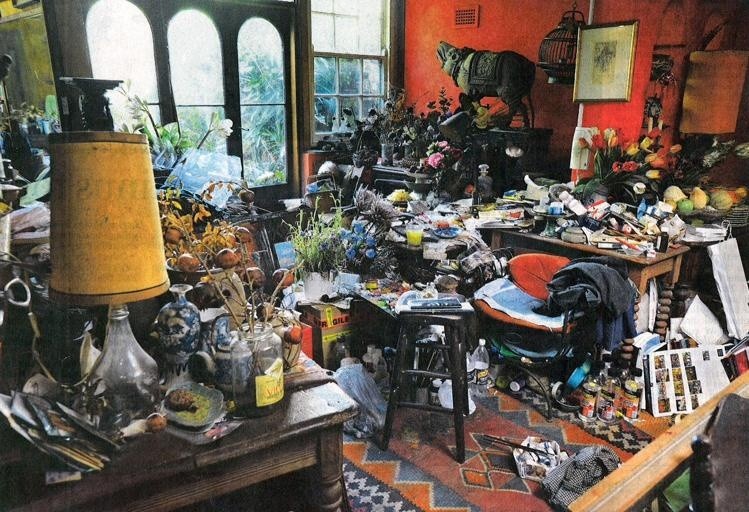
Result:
[297,299,377,369]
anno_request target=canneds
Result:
[581,380,640,421]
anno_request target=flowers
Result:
[572,123,684,201]
[281,190,401,273]
[426,139,455,170]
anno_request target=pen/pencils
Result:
[615,237,640,252]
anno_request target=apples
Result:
[677,198,694,213]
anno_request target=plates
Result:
[723,203,749,228]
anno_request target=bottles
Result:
[230,325,285,417]
[429,380,442,407]
[472,339,490,397]
[333,336,396,372]
[465,346,475,399]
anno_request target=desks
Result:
[0,349,363,511]
[490,228,692,361]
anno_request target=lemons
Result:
[737,186,747,199]
[666,199,676,211]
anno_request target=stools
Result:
[381,313,469,464]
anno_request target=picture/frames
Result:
[572,18,641,103]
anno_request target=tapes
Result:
[552,358,593,400]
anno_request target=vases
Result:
[302,268,333,302]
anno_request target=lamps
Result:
[47,128,172,408]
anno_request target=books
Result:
[631,293,749,418]
[273,240,295,269]
[407,297,463,309]
[684,221,728,243]
[596,242,629,250]
[393,301,475,316]
[591,236,628,244]
[0,388,123,485]
[12,225,50,245]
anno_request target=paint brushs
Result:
[484,434,555,456]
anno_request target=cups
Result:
[405,222,423,247]
